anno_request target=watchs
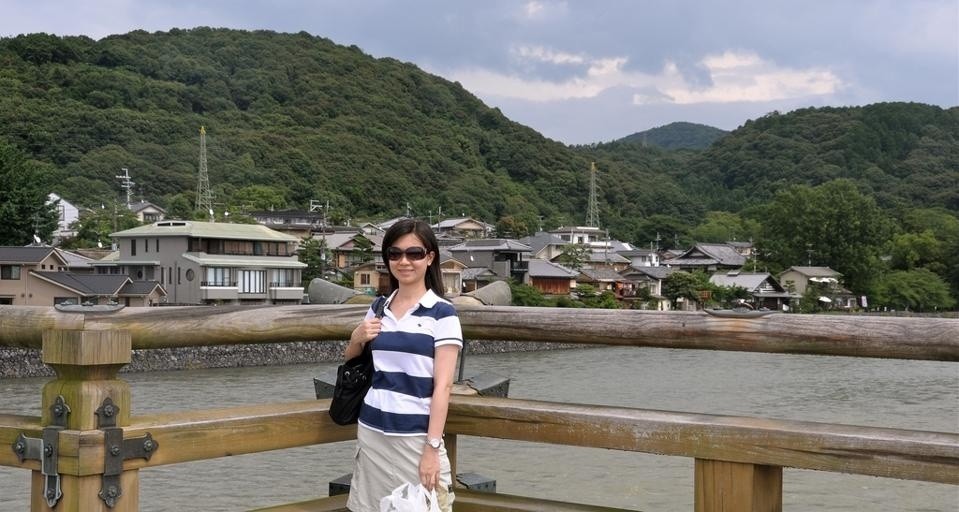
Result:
[426,439,441,449]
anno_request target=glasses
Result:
[385,246,429,261]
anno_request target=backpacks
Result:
[327,293,388,426]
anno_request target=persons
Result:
[346,219,463,511]
